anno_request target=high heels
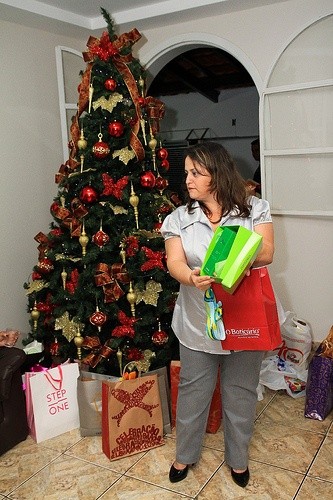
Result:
[230,466,250,488]
[169,460,195,483]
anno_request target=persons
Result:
[0,330,29,455]
[161,141,274,490]
[250,139,261,194]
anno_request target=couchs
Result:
[0,345,28,456]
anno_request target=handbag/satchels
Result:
[209,266,282,351]
[76,369,122,438]
[102,360,172,463]
[266,311,313,367]
[256,354,309,401]
[169,360,222,434]
[21,359,82,445]
[305,338,332,422]
